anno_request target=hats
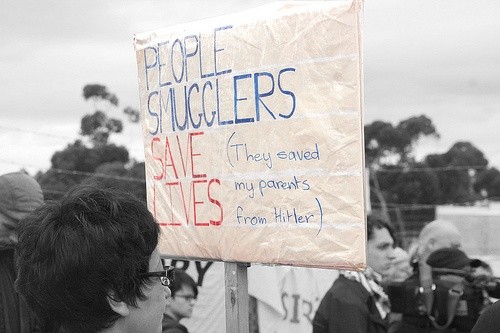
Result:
[1,174,46,223]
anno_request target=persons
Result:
[0,171,44,333]
[161,268,198,333]
[311,212,500,333]
[13,185,171,332]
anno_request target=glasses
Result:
[172,294,197,302]
[138,265,175,287]
